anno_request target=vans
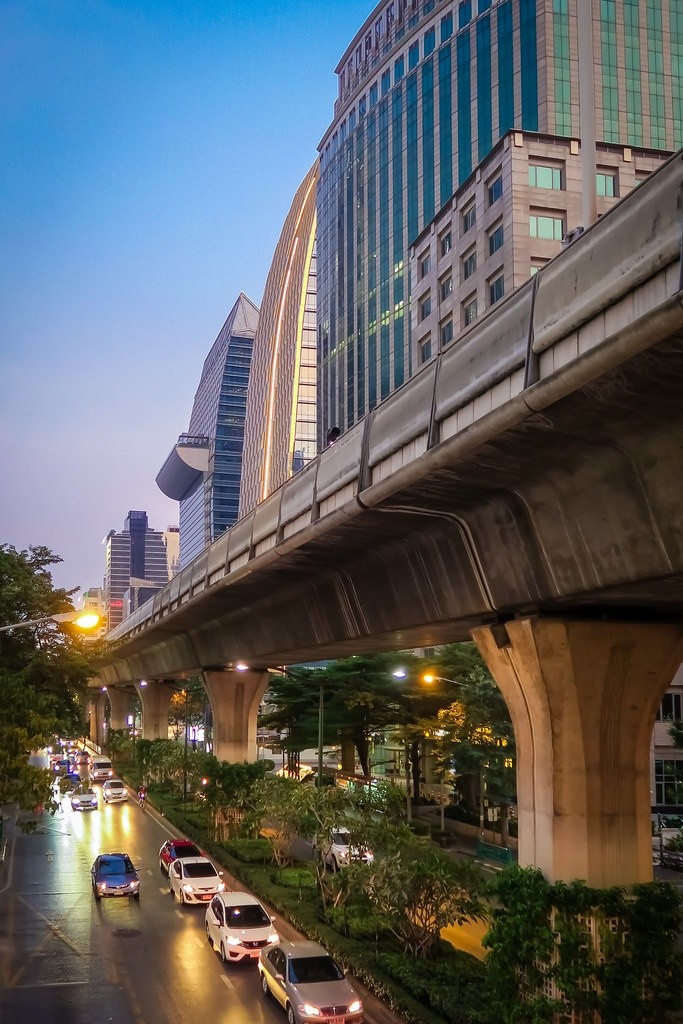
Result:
[88,755,113,783]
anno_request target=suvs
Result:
[311,826,375,874]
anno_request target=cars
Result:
[46,742,91,794]
[168,856,227,906]
[102,779,128,803]
[204,892,279,964]
[71,787,98,811]
[258,940,365,1024]
[90,853,141,902]
[158,840,204,873]
